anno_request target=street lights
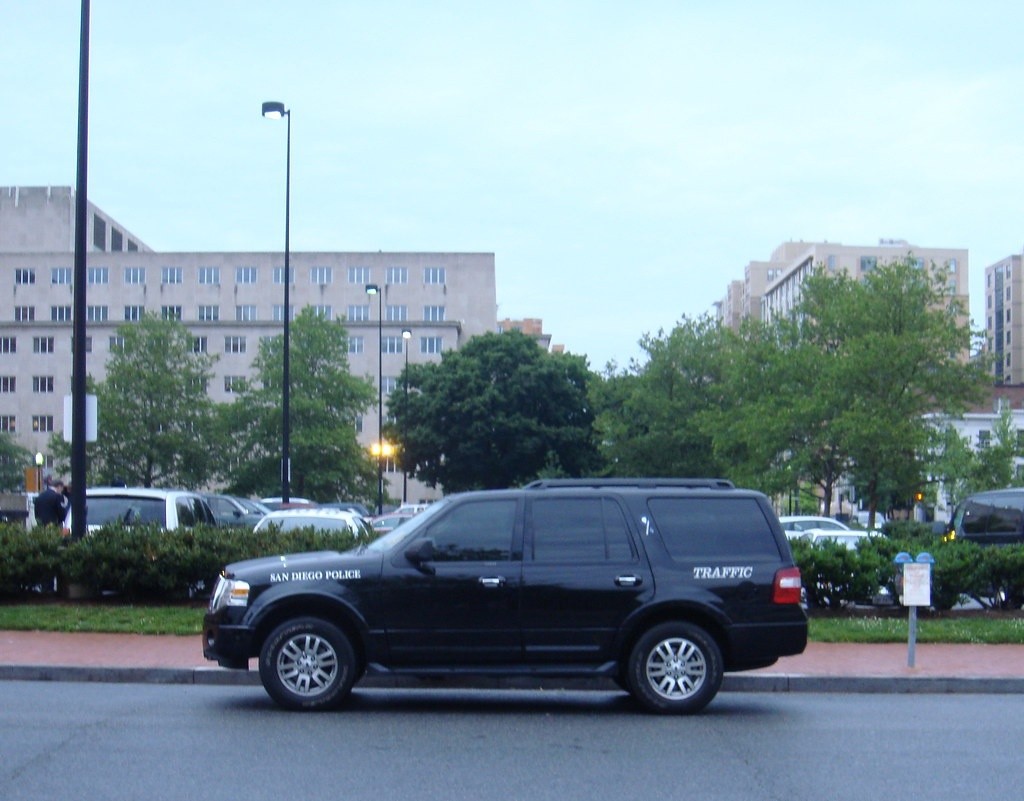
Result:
[365,284,382,515]
[402,330,412,503]
[36,452,44,493]
[262,102,291,505]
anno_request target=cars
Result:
[795,529,898,605]
[394,505,430,515]
[253,509,372,539]
[201,493,273,528]
[852,511,885,530]
[370,514,415,534]
[778,516,850,539]
[936,488,1024,609]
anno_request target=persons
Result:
[32,477,71,535]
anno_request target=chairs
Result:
[450,521,682,557]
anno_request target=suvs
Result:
[202,479,809,714]
[63,488,218,538]
